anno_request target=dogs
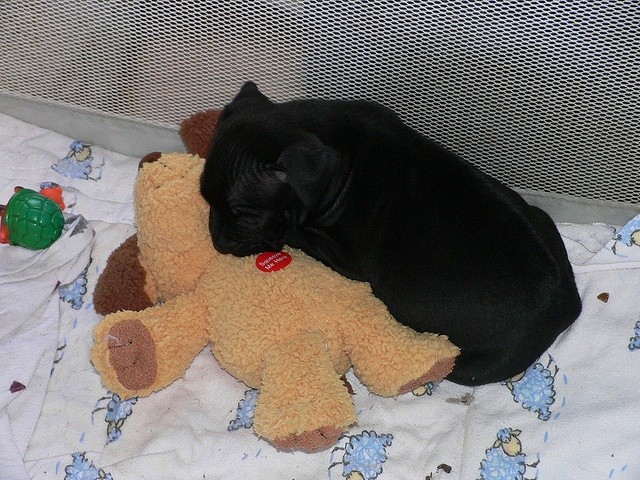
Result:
[198,81,583,387]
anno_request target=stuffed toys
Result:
[90,108,463,454]
